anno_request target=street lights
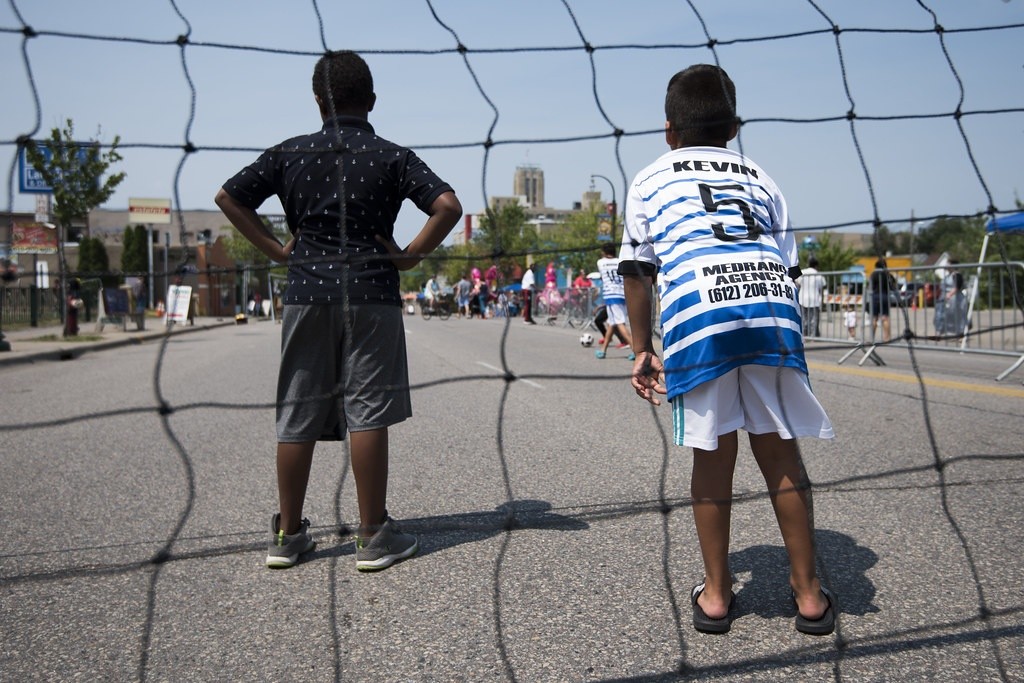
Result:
[589,174,617,256]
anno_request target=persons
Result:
[215,51,462,569]
[402,242,976,361]
[617,64,836,635]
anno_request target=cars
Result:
[887,281,938,307]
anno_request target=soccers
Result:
[579,333,594,349]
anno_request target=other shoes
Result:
[628,353,636,360]
[849,337,855,341]
[524,320,536,325]
[599,337,613,345]
[614,342,631,350]
[595,350,606,359]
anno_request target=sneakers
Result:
[354,509,418,570]
[266,513,316,566]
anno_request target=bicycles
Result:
[421,293,452,321]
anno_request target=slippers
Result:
[791,584,835,634]
[691,577,736,632]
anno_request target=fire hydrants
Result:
[62,294,83,337]
